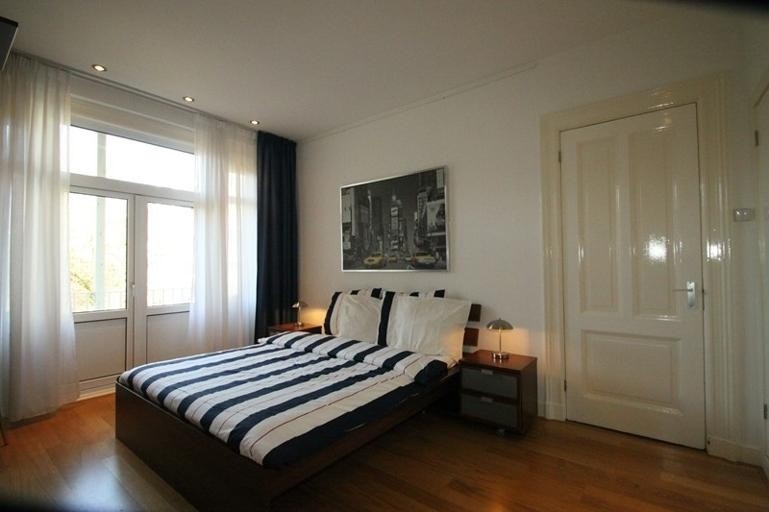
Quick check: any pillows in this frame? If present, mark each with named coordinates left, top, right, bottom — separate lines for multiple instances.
left=324, top=287, right=385, bottom=335
left=383, top=291, right=445, bottom=346
left=329, top=294, right=382, bottom=345
left=385, top=295, right=472, bottom=360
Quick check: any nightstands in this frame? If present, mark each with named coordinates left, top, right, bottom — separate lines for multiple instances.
left=266, top=322, right=322, bottom=336
left=460, top=349, right=536, bottom=436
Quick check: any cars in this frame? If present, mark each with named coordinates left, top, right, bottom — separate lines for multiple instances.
left=411, top=251, right=436, bottom=268
left=388, top=254, right=412, bottom=263
left=363, top=252, right=388, bottom=268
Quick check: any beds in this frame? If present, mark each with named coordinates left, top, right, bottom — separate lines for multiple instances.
left=116, top=304, right=482, bottom=508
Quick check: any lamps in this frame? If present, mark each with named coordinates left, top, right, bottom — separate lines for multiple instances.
left=293, top=302, right=308, bottom=326
left=486, top=317, right=514, bottom=360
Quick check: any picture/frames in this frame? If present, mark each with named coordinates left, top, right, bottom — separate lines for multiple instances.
left=339, top=165, right=450, bottom=274
